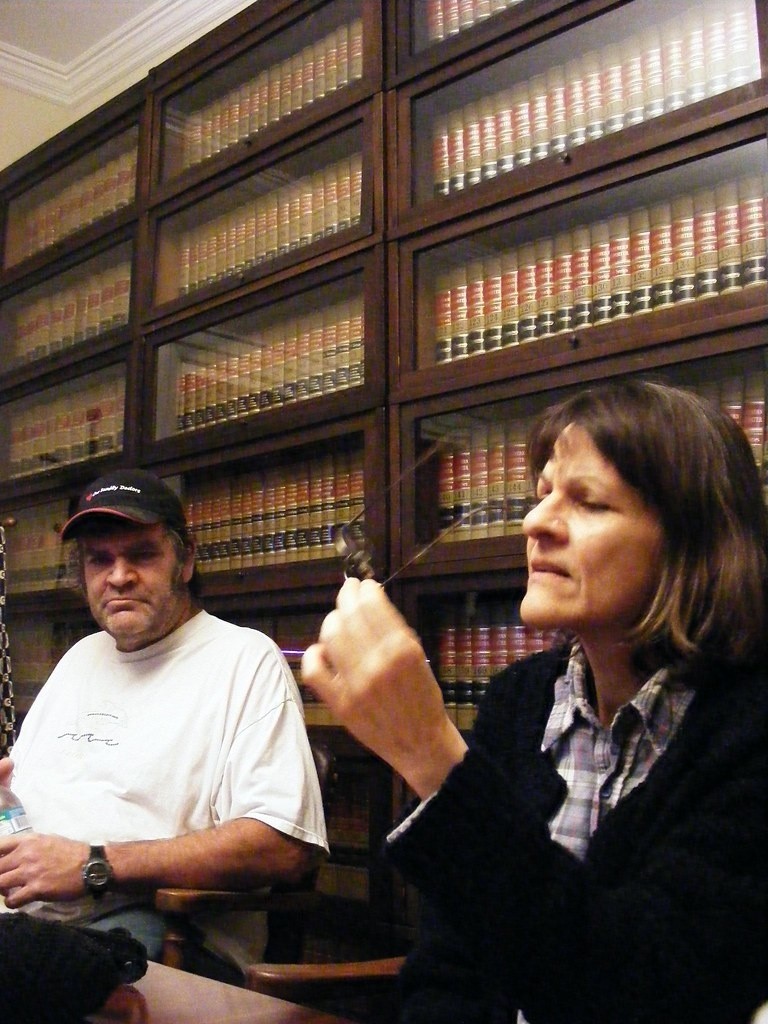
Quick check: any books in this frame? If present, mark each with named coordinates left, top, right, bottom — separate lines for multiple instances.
left=0, top=0, right=768, bottom=968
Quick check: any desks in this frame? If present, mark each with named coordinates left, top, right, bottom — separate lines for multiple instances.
left=125, top=960, right=359, bottom=1023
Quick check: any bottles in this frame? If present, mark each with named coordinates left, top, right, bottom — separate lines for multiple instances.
left=0, top=785, right=48, bottom=914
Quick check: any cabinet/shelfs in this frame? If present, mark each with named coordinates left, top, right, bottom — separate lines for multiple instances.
left=0, top=1, right=767, bottom=1024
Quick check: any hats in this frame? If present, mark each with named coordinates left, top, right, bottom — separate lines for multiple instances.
left=60, top=468, right=186, bottom=540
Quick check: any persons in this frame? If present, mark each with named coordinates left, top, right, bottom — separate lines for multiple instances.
left=300, top=379, right=768, bottom=1024
left=0, top=469, right=330, bottom=989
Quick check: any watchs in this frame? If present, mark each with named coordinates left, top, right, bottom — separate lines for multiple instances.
left=82, top=845, right=113, bottom=900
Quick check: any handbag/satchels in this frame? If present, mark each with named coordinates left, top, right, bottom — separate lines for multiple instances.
left=0, top=911, right=148, bottom=1024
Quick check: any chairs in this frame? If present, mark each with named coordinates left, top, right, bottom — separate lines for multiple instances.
left=156, top=739, right=341, bottom=970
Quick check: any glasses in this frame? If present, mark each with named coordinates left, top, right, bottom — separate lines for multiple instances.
left=331, top=431, right=514, bottom=590
